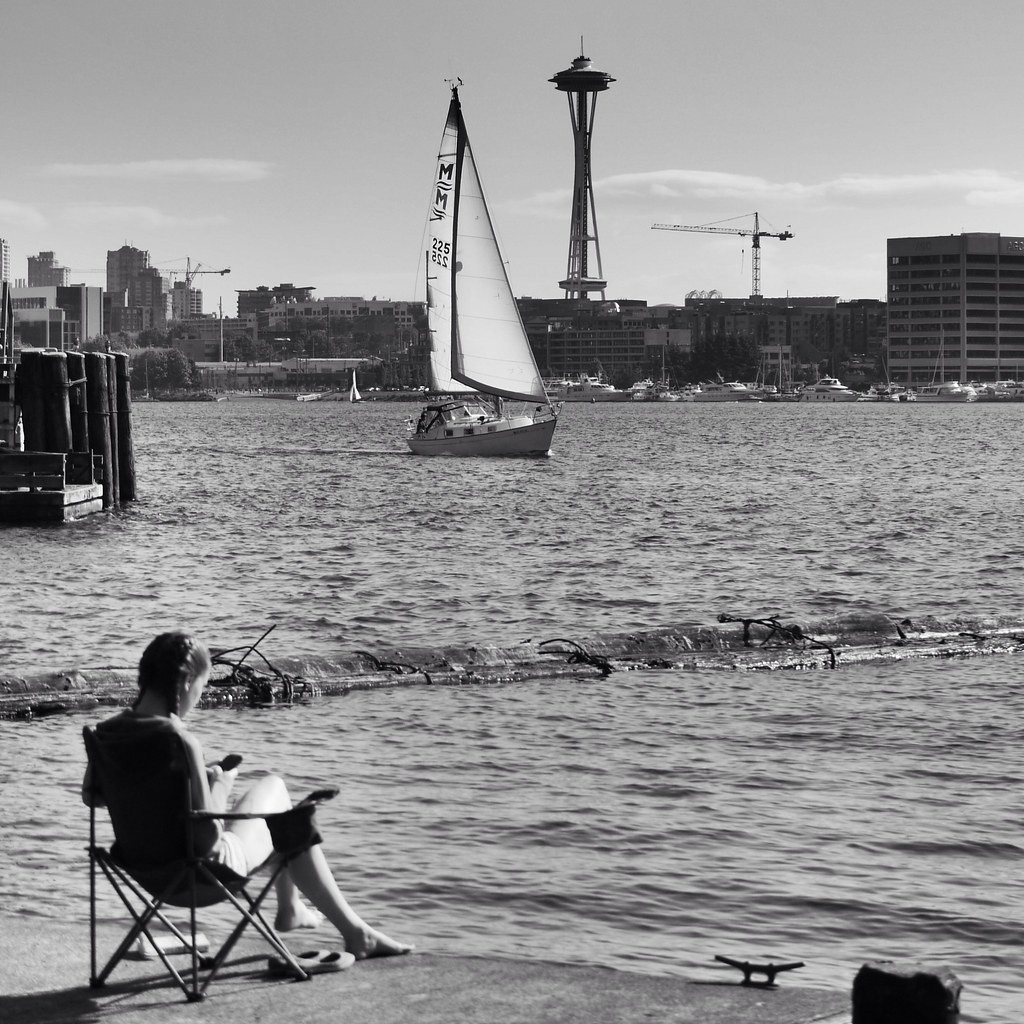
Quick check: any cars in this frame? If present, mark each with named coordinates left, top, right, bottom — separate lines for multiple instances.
left=364, top=385, right=430, bottom=393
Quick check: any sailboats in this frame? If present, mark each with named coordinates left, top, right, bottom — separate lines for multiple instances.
left=350, top=367, right=362, bottom=404
left=405, top=75, right=563, bottom=456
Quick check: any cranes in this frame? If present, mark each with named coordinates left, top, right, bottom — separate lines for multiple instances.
left=160, top=255, right=230, bottom=288
left=651, top=212, right=796, bottom=295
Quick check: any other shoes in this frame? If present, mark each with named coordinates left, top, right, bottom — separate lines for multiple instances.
left=267, top=949, right=331, bottom=977
left=300, top=951, right=355, bottom=973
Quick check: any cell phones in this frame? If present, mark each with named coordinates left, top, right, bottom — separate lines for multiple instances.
left=217, top=754, right=243, bottom=771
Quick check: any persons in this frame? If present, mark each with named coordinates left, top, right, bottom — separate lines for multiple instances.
left=417, top=413, right=428, bottom=433
left=495, top=397, right=504, bottom=417
left=79, top=631, right=415, bottom=958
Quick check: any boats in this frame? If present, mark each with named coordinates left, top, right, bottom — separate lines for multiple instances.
left=542, top=328, right=1024, bottom=403
left=296, top=392, right=321, bottom=402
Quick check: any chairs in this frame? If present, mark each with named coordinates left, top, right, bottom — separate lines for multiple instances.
left=79, top=724, right=341, bottom=1008
